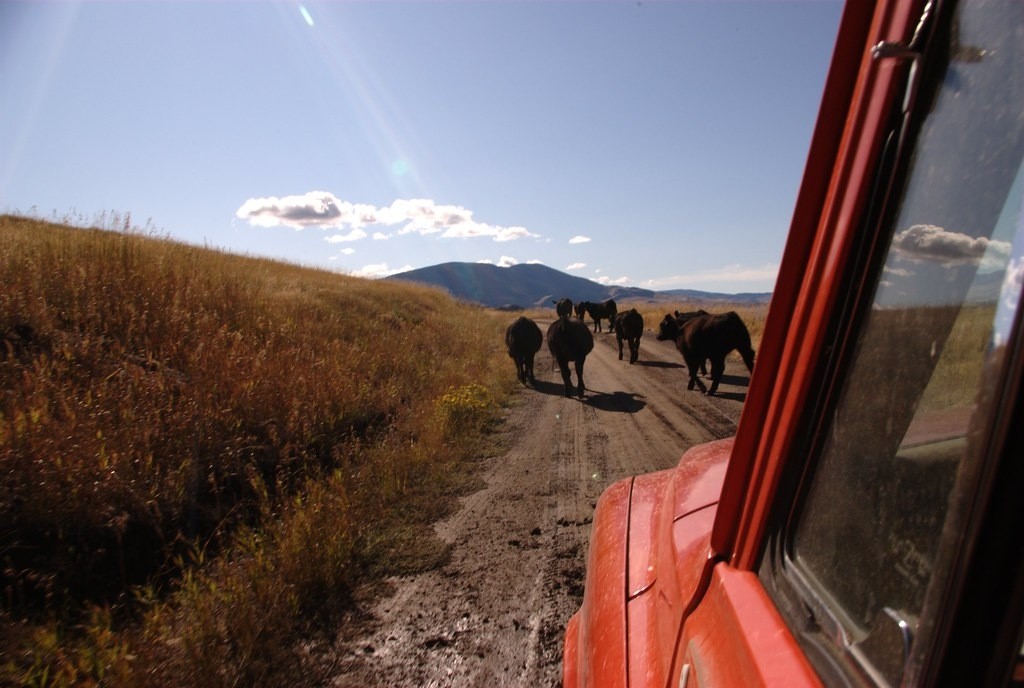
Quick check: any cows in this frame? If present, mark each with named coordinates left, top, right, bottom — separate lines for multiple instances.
left=506, top=299, right=756, bottom=397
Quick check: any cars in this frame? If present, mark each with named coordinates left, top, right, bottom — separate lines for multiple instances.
left=557, top=0, right=1023, bottom=688
